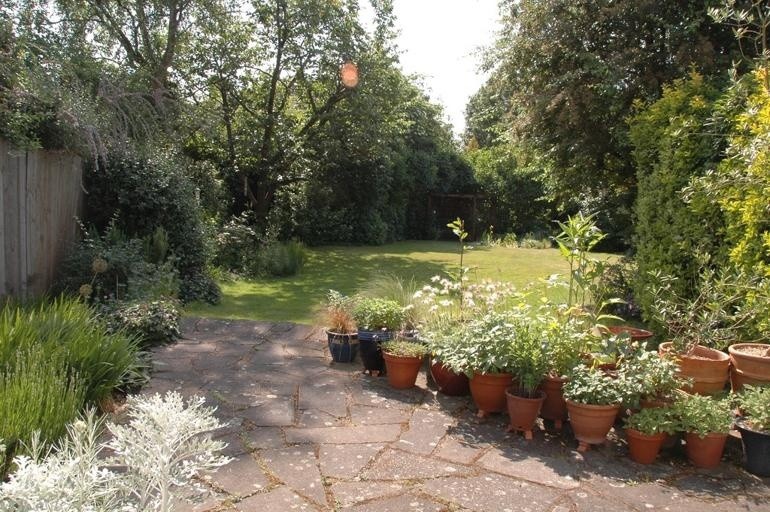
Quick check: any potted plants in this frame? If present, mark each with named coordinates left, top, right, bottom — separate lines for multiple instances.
left=539, top=213, right=769, bottom=452
left=323, top=290, right=361, bottom=362
left=351, top=294, right=406, bottom=376
left=406, top=217, right=546, bottom=441
left=622, top=400, right=677, bottom=465
left=728, top=381, right=769, bottom=477
left=677, top=391, right=732, bottom=471
left=378, top=327, right=428, bottom=389
